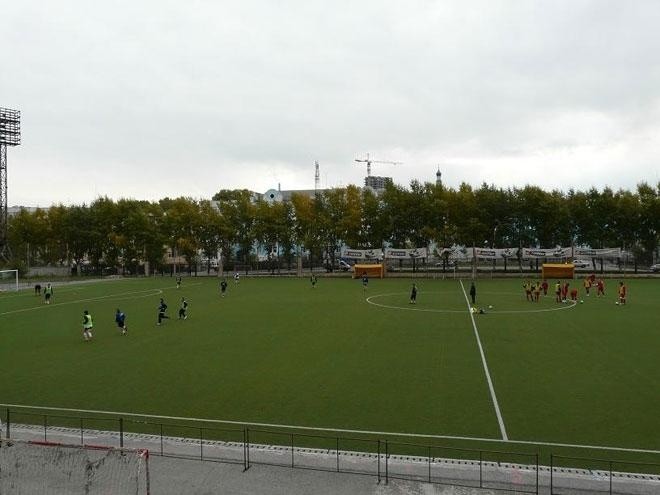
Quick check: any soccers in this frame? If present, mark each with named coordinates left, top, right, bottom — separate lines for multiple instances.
left=489, top=304, right=493, bottom=309
left=615, top=301, right=619, bottom=305
left=580, top=300, right=583, bottom=304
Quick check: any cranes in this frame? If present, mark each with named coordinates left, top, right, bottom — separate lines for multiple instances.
left=353, top=150, right=400, bottom=191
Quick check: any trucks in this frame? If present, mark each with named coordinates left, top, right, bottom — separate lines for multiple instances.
left=322, top=259, right=351, bottom=271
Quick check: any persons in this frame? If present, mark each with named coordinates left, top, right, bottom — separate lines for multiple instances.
left=83, top=311, right=94, bottom=342
left=35, top=284, right=41, bottom=296
left=177, top=297, right=187, bottom=320
left=410, top=284, right=418, bottom=304
left=469, top=281, right=476, bottom=304
left=44, top=283, right=53, bottom=305
left=233, top=272, right=240, bottom=287
left=523, top=272, right=626, bottom=305
left=311, top=274, right=315, bottom=287
left=220, top=278, right=227, bottom=298
left=115, top=307, right=127, bottom=336
left=175, top=272, right=181, bottom=288
left=157, top=299, right=170, bottom=326
left=362, top=271, right=368, bottom=286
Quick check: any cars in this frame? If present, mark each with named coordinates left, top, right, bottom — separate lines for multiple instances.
left=435, top=260, right=455, bottom=268
left=571, top=260, right=590, bottom=268
